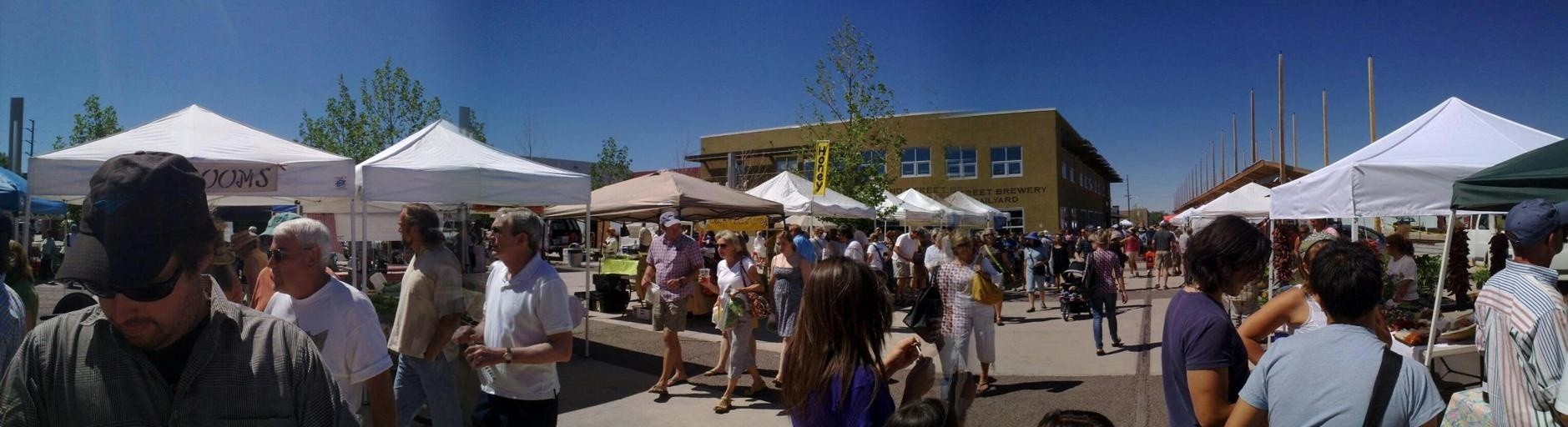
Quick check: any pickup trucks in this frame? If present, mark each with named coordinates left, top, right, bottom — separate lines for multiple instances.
left=1463, top=214, right=1513, bottom=264
left=1393, top=217, right=1424, bottom=231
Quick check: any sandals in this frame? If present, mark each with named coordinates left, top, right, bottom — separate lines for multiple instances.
left=744, top=382, right=768, bottom=394
left=714, top=396, right=733, bottom=412
left=997, top=320, right=1004, bottom=326
left=1026, top=307, right=1035, bottom=312
left=1040, top=303, right=1047, bottom=308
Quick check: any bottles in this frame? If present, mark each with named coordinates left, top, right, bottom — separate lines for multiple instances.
left=466, top=340, right=500, bottom=387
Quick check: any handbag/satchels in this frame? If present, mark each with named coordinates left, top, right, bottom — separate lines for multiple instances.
left=903, top=265, right=944, bottom=344
left=1003, top=269, right=1012, bottom=278
left=748, top=292, right=770, bottom=319
left=1033, top=265, right=1046, bottom=276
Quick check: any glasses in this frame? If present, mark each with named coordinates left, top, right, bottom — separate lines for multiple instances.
left=267, top=248, right=310, bottom=260
left=715, top=243, right=734, bottom=249
left=80, top=259, right=188, bottom=302
left=985, top=233, right=993, bottom=236
left=1385, top=243, right=1390, bottom=247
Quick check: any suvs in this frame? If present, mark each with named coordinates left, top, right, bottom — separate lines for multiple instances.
left=545, top=217, right=582, bottom=256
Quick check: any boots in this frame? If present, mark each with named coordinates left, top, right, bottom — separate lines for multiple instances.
left=704, top=370, right=728, bottom=375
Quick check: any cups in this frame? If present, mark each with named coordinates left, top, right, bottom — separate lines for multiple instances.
left=700, top=269, right=710, bottom=285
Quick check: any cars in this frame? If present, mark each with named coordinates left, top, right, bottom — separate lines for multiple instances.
left=342, top=241, right=388, bottom=263
left=1336, top=224, right=1387, bottom=247
left=19, top=221, right=34, bottom=241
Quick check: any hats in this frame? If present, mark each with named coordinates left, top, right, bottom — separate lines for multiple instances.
left=211, top=244, right=236, bottom=265
left=659, top=211, right=682, bottom=228
left=228, top=231, right=258, bottom=250
left=257, top=211, right=303, bottom=236
left=54, top=150, right=206, bottom=283
left=915, top=227, right=925, bottom=235
left=1085, top=224, right=1098, bottom=231
left=607, top=227, right=616, bottom=235
left=1024, top=231, right=1041, bottom=241
left=1504, top=198, right=1568, bottom=245
left=1044, top=230, right=1049, bottom=237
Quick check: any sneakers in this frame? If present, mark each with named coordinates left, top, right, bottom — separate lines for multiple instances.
left=1172, top=271, right=1178, bottom=276
left=1154, top=284, right=1160, bottom=289
left=1163, top=285, right=1169, bottom=289
left=1129, top=275, right=1135, bottom=278
left=1135, top=272, right=1139, bottom=277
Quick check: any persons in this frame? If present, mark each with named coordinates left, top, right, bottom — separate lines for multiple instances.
left=0, top=148, right=571, bottom=427
left=642, top=212, right=704, bottom=395
left=1161, top=199, right=1568, bottom=425
left=602, top=222, right=1157, bottom=427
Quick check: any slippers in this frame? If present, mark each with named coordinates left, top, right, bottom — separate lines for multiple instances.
left=977, top=382, right=990, bottom=393
left=1111, top=338, right=1121, bottom=345
left=649, top=387, right=669, bottom=396
left=1097, top=350, right=1105, bottom=356
left=668, top=375, right=686, bottom=386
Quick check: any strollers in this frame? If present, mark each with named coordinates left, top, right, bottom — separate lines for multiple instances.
left=1059, top=257, right=1095, bottom=321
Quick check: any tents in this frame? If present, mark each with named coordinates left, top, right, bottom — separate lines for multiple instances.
left=542, top=169, right=787, bottom=259
left=0, top=103, right=356, bottom=288
left=296, top=118, right=590, bottom=358
left=876, top=187, right=1007, bottom=227
left=1159, top=182, right=1270, bottom=235
left=1268, top=96, right=1568, bottom=366
left=692, top=170, right=876, bottom=240
